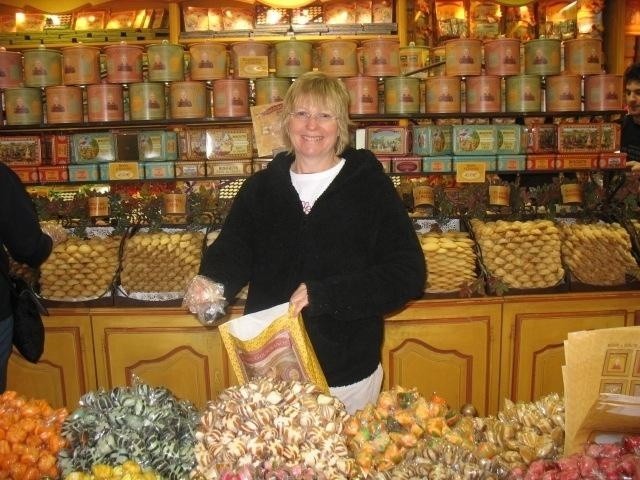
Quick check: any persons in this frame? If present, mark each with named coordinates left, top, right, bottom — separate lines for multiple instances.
left=619, top=60, right=640, bottom=179
left=1, top=159, right=70, bottom=392
left=182, top=72, right=429, bottom=418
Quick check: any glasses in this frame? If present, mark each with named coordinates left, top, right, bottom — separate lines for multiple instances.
left=290, top=111, right=336, bottom=121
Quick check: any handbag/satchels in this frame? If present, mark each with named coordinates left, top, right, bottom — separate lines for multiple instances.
left=9, top=275, right=48, bottom=363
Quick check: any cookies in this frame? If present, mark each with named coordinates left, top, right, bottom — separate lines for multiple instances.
left=0, top=217, right=640, bottom=299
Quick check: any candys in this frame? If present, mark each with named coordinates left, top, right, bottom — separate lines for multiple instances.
left=0, top=379, right=639, bottom=480
